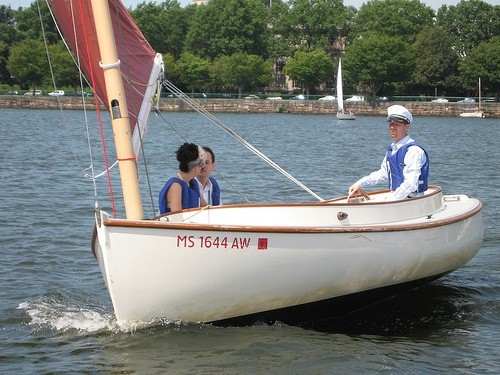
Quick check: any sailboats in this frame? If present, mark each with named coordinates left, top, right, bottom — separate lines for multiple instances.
left=33, top=0, right=485, bottom=335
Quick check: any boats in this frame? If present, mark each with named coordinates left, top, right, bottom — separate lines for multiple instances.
left=336, top=112, right=356, bottom=120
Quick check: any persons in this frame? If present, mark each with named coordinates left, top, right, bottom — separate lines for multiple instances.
left=187, top=147, right=222, bottom=207
left=348, top=105, right=429, bottom=202
left=159, top=143, right=208, bottom=214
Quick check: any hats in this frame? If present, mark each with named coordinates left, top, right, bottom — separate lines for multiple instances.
left=386, top=105, right=413, bottom=124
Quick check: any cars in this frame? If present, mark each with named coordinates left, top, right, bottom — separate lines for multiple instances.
left=4, top=89, right=475, bottom=105
